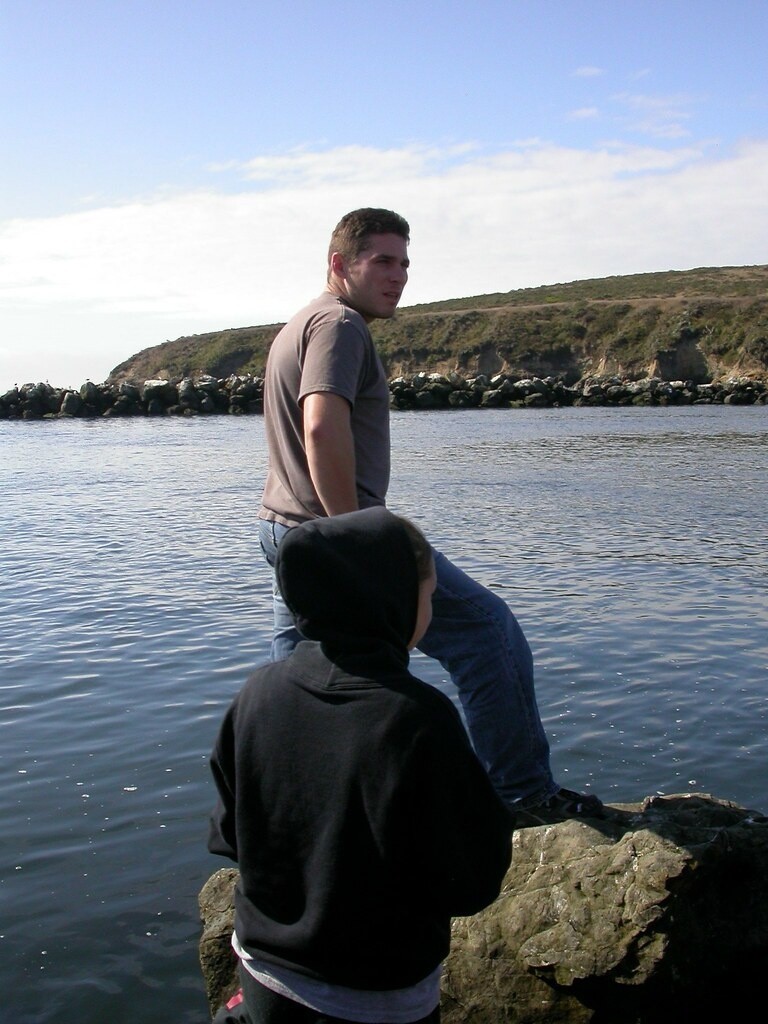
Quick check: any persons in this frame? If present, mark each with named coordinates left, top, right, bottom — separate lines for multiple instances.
left=206, top=506, right=523, bottom=1023
left=255, top=205, right=605, bottom=829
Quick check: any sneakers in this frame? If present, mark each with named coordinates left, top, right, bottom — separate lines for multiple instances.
left=511, top=788, right=603, bottom=829
